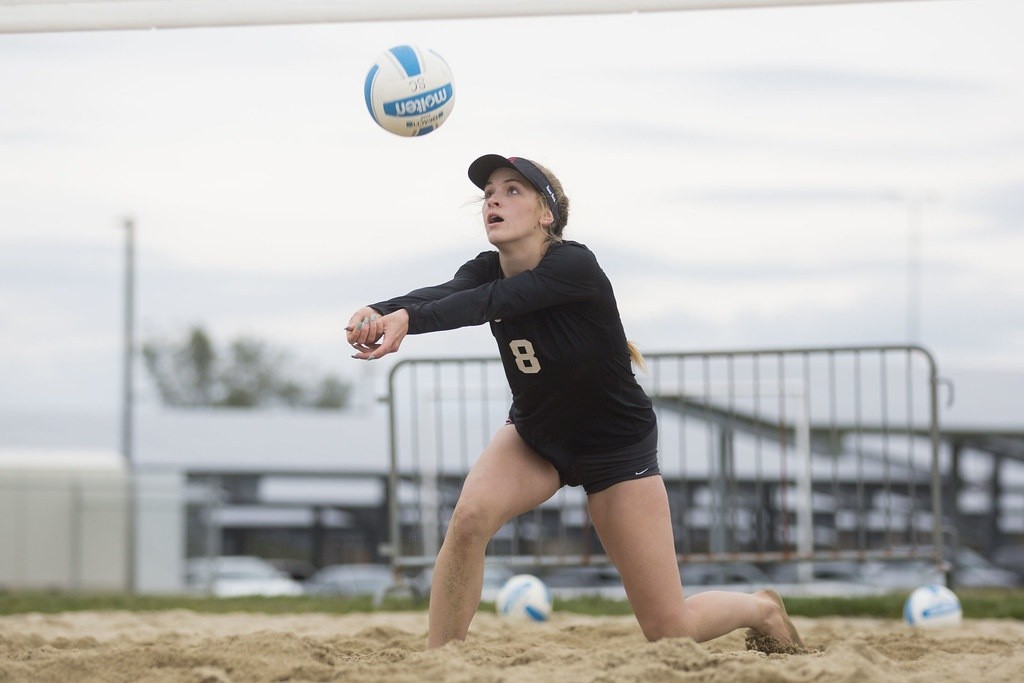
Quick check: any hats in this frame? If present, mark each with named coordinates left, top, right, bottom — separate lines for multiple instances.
left=466, top=153, right=563, bottom=229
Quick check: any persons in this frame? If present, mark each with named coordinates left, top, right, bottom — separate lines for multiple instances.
left=343, top=153, right=806, bottom=659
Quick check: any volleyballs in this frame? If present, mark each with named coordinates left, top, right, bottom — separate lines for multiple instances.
left=364, top=45, right=455, bottom=136
left=496, top=574, right=553, bottom=623
left=900, top=584, right=962, bottom=628
